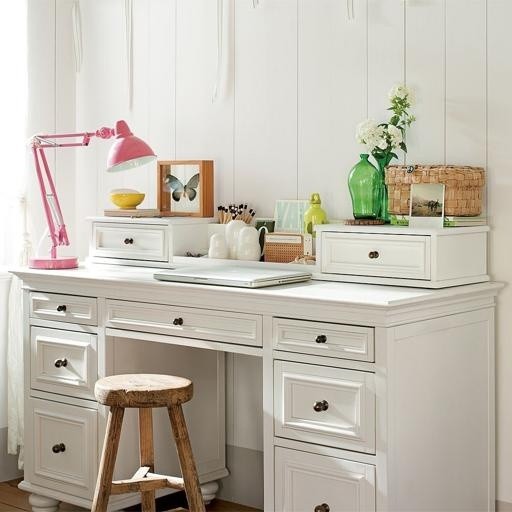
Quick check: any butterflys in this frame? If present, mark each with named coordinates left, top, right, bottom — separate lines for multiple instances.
left=163, top=172, right=200, bottom=203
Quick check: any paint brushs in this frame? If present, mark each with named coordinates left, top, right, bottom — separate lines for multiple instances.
left=217, top=202, right=256, bottom=224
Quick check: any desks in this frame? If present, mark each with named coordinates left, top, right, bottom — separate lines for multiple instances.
left=8, top=217, right=506, bottom=512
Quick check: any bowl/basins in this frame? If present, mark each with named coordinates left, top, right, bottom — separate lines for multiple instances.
left=111, top=192, right=145, bottom=210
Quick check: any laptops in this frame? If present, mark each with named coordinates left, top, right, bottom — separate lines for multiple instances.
left=154, top=270, right=313, bottom=288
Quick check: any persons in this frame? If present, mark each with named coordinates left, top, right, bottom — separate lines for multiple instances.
left=428, top=199, right=440, bottom=214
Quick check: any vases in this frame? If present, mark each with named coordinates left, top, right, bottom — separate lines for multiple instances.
left=370, top=153, right=395, bottom=219
left=347, top=153, right=382, bottom=219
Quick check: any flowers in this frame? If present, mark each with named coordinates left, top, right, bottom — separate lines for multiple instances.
left=353, top=82, right=417, bottom=159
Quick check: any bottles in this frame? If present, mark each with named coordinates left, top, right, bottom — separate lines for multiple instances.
left=304, top=193, right=328, bottom=261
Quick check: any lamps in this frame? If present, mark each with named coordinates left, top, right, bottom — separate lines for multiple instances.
left=26, top=119, right=158, bottom=270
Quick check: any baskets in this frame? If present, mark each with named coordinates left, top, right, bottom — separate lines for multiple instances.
left=263, top=232, right=304, bottom=263
left=384, top=163, right=486, bottom=216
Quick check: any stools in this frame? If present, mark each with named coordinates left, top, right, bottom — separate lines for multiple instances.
left=89, top=371, right=207, bottom=512
